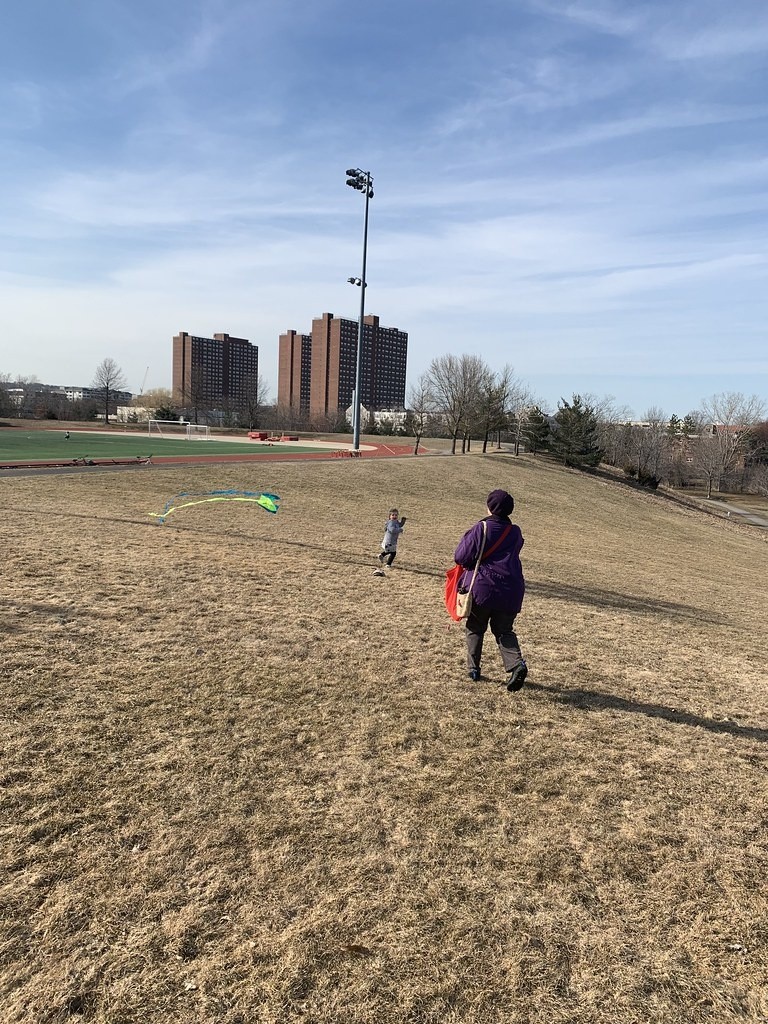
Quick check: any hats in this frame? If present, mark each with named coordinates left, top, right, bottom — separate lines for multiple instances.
left=487, top=489, right=514, bottom=515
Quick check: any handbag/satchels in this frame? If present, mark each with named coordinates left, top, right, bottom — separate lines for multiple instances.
left=446, top=564, right=463, bottom=622
left=456, top=588, right=472, bottom=617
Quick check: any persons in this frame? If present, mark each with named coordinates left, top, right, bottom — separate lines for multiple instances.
left=64, top=431, right=70, bottom=439
left=379, top=509, right=406, bottom=567
left=454, top=489, right=528, bottom=691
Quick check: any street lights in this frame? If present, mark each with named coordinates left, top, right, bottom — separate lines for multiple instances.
left=345, top=167, right=375, bottom=448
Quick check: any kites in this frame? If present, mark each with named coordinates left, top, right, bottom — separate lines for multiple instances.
left=148, top=490, right=281, bottom=522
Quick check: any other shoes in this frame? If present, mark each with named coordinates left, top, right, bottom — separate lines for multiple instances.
left=384, top=564, right=392, bottom=571
left=507, top=664, right=527, bottom=693
left=468, top=668, right=480, bottom=680
left=378, top=554, right=383, bottom=563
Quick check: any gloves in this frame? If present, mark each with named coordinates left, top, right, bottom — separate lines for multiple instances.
left=401, top=517, right=406, bottom=523
left=399, top=529, right=403, bottom=533
